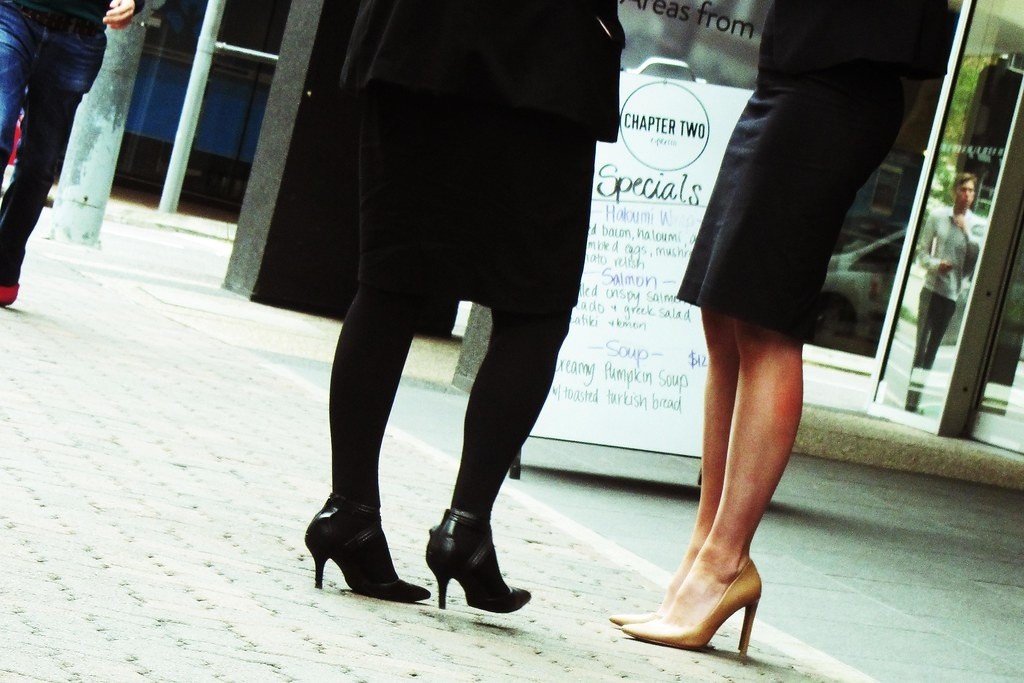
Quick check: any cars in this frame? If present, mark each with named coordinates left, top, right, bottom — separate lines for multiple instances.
left=815, top=214, right=1024, bottom=384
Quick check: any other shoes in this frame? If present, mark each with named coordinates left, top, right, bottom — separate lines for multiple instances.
left=0, top=282, right=20, bottom=305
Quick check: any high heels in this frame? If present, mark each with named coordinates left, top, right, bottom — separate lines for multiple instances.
left=620, top=557, right=762, bottom=657
left=609, top=610, right=668, bottom=627
left=304, top=496, right=431, bottom=601
left=424, top=508, right=531, bottom=613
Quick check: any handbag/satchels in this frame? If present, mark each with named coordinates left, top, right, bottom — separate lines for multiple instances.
left=338, top=0, right=630, bottom=143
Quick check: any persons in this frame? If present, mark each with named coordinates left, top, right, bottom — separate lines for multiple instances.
left=0, top=0, right=145, bottom=305
left=905, top=172, right=978, bottom=412
left=305, top=0, right=622, bottom=613
left=609, top=0, right=942, bottom=654
